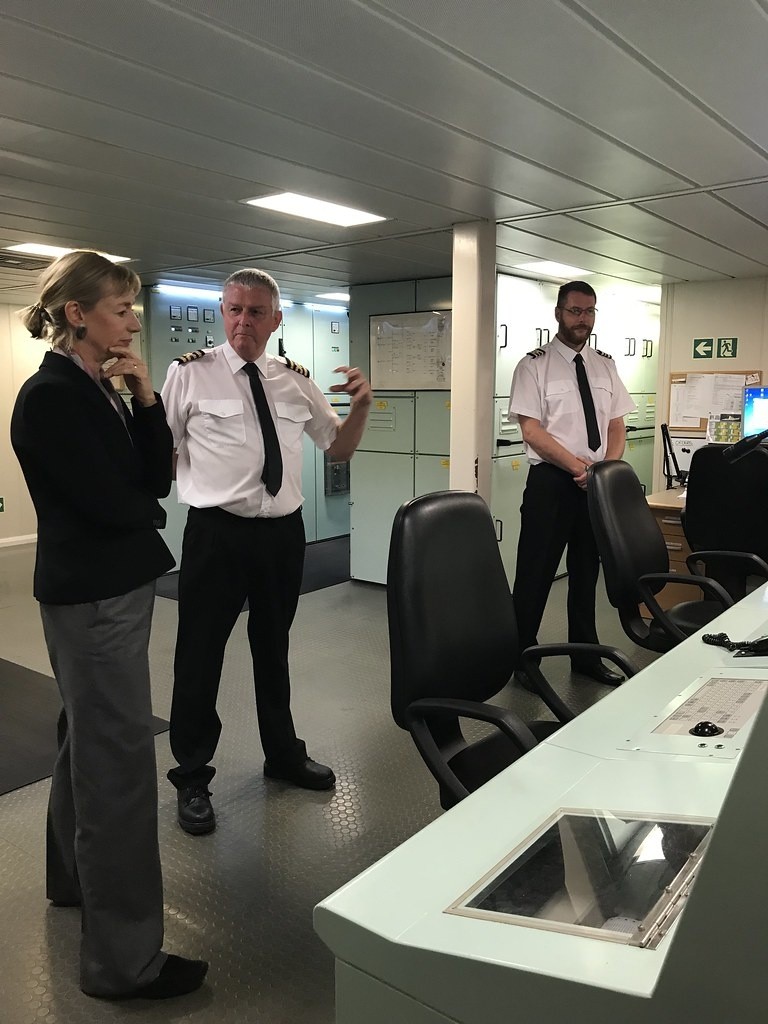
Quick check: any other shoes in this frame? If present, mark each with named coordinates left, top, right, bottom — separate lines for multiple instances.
left=52, top=898, right=82, bottom=907
left=82, top=954, right=209, bottom=1000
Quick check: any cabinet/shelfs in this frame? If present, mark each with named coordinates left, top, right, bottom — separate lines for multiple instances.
left=121, top=279, right=451, bottom=589
left=637, top=485, right=707, bottom=620
left=497, top=274, right=661, bottom=595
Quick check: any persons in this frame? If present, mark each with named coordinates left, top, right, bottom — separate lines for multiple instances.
left=11, top=251, right=210, bottom=1003
left=161, top=268, right=373, bottom=837
left=507, top=280, right=638, bottom=695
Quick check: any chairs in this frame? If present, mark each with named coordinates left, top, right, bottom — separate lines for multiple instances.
left=585, top=458, right=768, bottom=657
left=381, top=487, right=642, bottom=819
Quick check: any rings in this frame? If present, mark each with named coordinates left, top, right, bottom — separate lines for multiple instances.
left=133, top=363, right=137, bottom=368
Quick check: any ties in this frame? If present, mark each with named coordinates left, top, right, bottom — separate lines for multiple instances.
left=573, top=354, right=601, bottom=452
left=242, top=363, right=283, bottom=497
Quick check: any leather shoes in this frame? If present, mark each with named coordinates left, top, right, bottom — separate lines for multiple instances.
left=570, top=660, right=625, bottom=687
left=513, top=668, right=541, bottom=697
left=264, top=754, right=337, bottom=789
left=177, top=782, right=216, bottom=835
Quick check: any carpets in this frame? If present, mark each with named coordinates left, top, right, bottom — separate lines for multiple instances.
left=0, top=658, right=170, bottom=797
left=155, top=535, right=354, bottom=614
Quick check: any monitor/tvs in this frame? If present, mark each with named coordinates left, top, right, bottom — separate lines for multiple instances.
left=741, top=385, right=768, bottom=446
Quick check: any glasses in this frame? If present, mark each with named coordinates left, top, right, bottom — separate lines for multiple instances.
left=557, top=305, right=598, bottom=317
